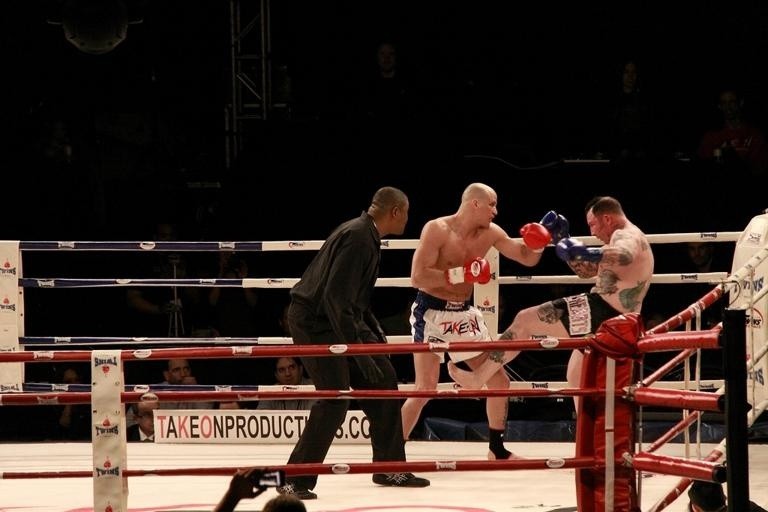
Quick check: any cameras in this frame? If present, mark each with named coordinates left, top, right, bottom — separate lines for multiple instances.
left=255, top=468, right=283, bottom=489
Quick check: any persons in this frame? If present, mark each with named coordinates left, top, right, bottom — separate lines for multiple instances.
left=445, top=195, right=654, bottom=423
left=210, top=467, right=308, bottom=512
left=275, top=184, right=434, bottom=501
left=397, top=181, right=554, bottom=461
left=1, top=3, right=768, bottom=444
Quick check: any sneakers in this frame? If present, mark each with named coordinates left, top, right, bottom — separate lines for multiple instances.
left=372, top=468, right=432, bottom=489
left=275, top=472, right=319, bottom=501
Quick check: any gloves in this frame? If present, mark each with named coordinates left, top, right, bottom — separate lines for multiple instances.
left=374, top=324, right=392, bottom=359
left=445, top=254, right=492, bottom=287
left=537, top=210, right=571, bottom=247
left=517, top=220, right=552, bottom=254
left=555, top=236, right=604, bottom=264
left=352, top=344, right=387, bottom=387
